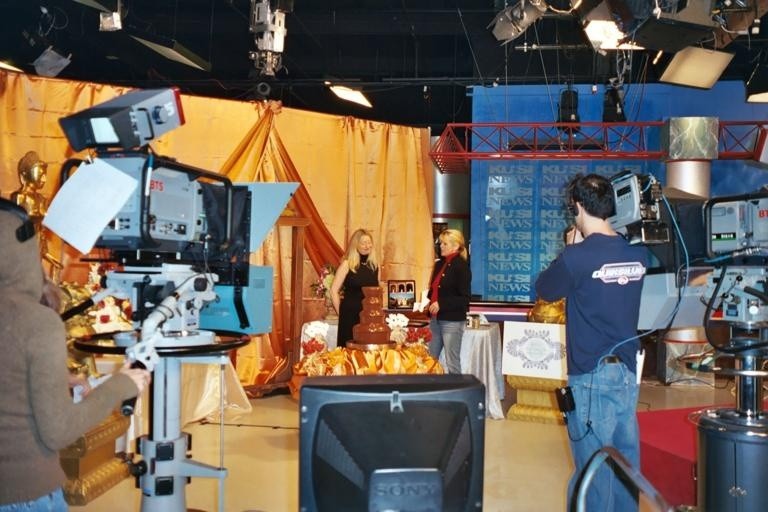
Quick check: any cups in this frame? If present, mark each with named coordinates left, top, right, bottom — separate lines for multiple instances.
left=472, top=319, right=481, bottom=329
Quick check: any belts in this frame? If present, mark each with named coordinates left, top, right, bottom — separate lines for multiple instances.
left=600, top=354, right=623, bottom=365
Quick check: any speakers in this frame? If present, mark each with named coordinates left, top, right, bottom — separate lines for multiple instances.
left=602, top=89, right=627, bottom=123
left=557, top=90, right=581, bottom=136
left=296, top=373, right=486, bottom=512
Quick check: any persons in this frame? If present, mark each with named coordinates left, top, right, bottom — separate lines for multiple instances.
left=329, top=228, right=384, bottom=350
left=533, top=173, right=647, bottom=511
left=423, top=228, right=473, bottom=375
left=1, top=194, right=153, bottom=512
left=10, top=152, right=65, bottom=271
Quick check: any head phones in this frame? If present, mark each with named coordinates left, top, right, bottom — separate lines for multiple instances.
left=571, top=176, right=586, bottom=216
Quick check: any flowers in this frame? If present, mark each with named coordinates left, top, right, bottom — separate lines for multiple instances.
left=313, top=264, right=335, bottom=300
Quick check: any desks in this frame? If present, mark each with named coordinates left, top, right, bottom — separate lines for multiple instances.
left=299, top=319, right=506, bottom=423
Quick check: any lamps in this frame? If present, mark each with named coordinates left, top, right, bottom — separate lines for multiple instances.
left=486, top=2, right=768, bottom=133
left=119, top=0, right=216, bottom=73
